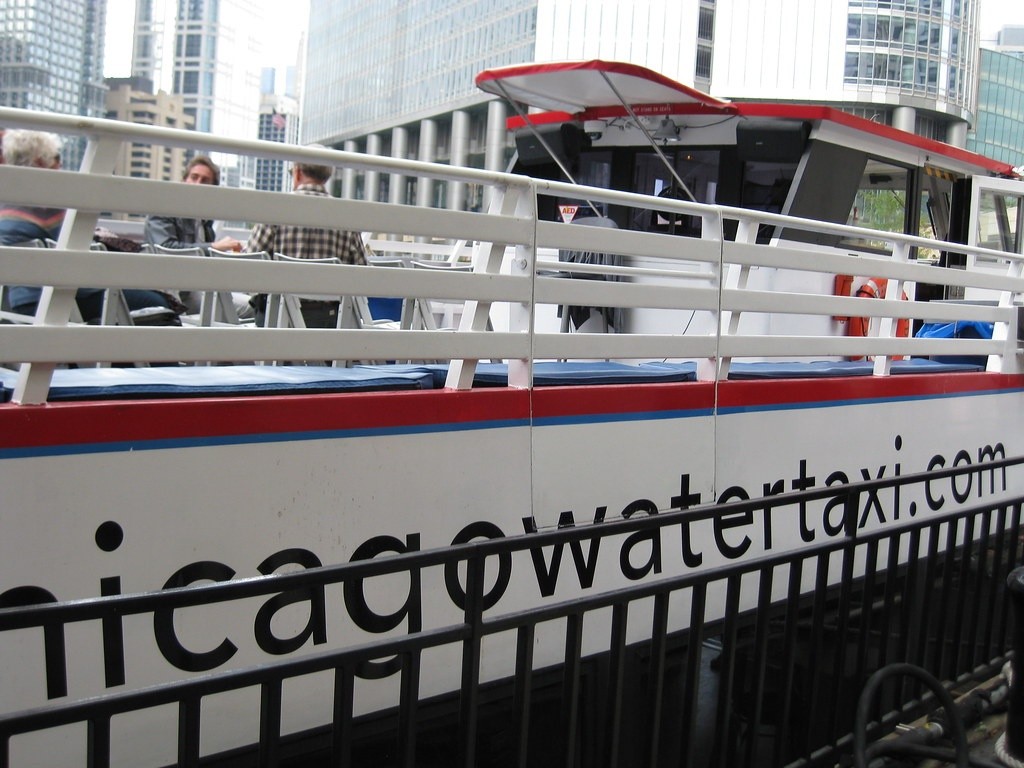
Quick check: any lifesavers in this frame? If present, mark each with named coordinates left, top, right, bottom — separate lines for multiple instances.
left=848, top=276, right=912, bottom=364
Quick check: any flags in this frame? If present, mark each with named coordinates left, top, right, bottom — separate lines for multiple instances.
left=273, top=107, right=287, bottom=130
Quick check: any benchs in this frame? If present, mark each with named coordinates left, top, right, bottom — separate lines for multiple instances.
left=0, top=359, right=985, bottom=403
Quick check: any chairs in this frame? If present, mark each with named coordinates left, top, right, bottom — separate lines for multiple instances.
left=10, top=237, right=503, bottom=368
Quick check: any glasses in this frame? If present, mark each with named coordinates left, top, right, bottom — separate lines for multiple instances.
left=288, top=168, right=296, bottom=176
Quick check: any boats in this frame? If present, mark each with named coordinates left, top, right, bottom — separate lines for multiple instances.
left=0, top=60, right=1023, bottom=767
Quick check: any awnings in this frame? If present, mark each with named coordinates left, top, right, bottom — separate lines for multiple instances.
left=475, top=59, right=736, bottom=217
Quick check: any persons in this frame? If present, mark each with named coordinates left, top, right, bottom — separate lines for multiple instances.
left=241, top=144, right=365, bottom=329
left=144, top=155, right=256, bottom=318
left=0, top=128, right=190, bottom=368
